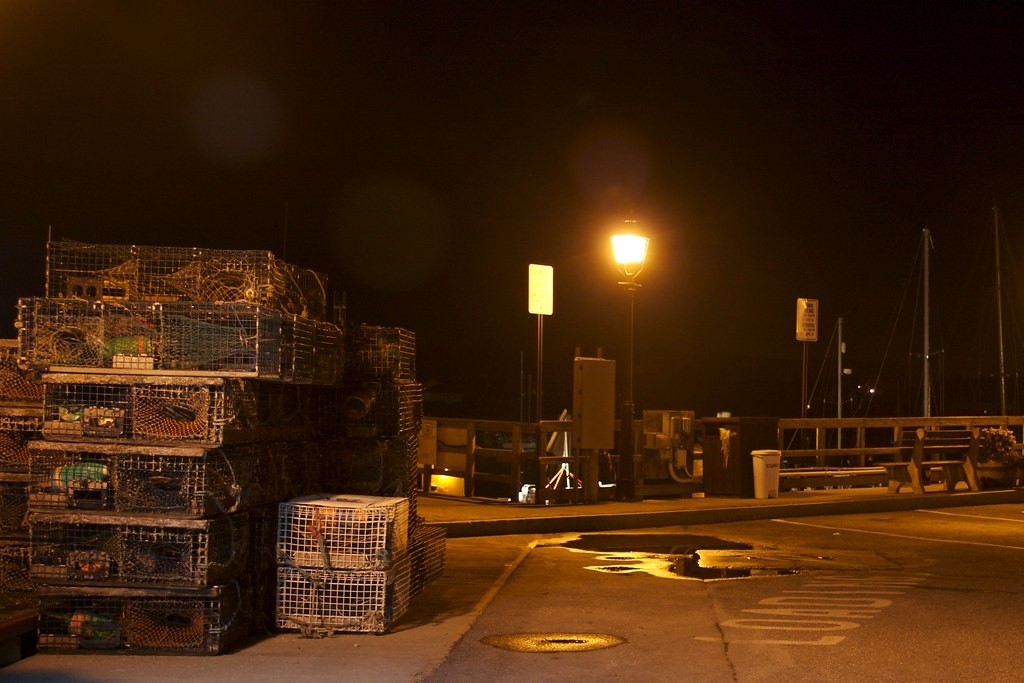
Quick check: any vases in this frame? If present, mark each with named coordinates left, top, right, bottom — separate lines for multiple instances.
left=975, top=462, right=1017, bottom=489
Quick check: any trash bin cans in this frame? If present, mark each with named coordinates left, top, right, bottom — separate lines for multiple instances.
left=750, top=449, right=781, bottom=499
left=700, top=416, right=778, bottom=499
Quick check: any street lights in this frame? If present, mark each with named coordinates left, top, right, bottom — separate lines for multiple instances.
left=609, top=203, right=651, bottom=502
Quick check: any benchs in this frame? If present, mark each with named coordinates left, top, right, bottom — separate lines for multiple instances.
left=879, top=427, right=984, bottom=495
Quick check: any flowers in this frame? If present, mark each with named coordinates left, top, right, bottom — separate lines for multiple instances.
left=978, top=427, right=1024, bottom=462
